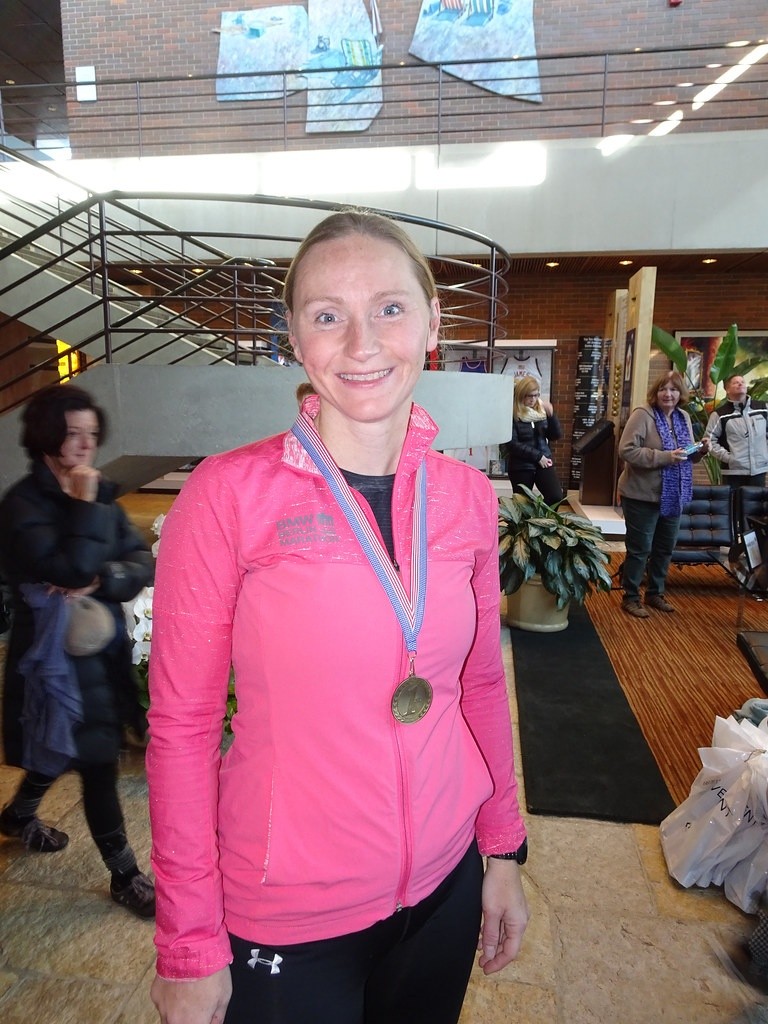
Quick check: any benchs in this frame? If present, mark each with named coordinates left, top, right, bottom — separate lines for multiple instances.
left=670, top=484, right=768, bottom=601
left=736, top=630, right=768, bottom=696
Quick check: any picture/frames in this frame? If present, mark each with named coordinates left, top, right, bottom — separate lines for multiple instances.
left=672, top=330, right=768, bottom=422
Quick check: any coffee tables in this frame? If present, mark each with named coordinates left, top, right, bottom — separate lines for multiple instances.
left=706, top=549, right=768, bottom=601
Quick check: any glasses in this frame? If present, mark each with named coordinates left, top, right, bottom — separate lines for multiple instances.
left=525, top=393, right=541, bottom=399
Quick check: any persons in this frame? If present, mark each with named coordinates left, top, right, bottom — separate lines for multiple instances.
left=497, top=375, right=563, bottom=508
left=0, top=385, right=157, bottom=921
left=702, top=375, right=768, bottom=486
left=616, top=371, right=713, bottom=618
left=146, top=209, right=532, bottom=1024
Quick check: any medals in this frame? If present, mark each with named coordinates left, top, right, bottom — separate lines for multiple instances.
left=391, top=659, right=433, bottom=725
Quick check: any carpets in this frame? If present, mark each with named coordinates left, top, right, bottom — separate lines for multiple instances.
left=0, top=622, right=768, bottom=1022
left=510, top=596, right=677, bottom=822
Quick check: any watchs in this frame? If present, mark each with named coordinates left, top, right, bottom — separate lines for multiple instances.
left=489, top=835, right=530, bottom=865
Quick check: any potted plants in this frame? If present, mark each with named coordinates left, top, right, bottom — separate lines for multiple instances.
left=497, top=483, right=614, bottom=631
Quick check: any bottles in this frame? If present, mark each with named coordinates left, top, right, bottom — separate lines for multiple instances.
left=676, top=442, right=704, bottom=457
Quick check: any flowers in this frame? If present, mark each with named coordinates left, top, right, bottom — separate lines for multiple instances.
left=118, top=514, right=239, bottom=722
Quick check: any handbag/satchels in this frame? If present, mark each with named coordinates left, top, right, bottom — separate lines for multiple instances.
left=499, top=443, right=509, bottom=460
left=61, top=593, right=115, bottom=656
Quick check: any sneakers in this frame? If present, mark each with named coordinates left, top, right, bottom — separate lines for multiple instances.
left=643, top=593, right=675, bottom=611
left=621, top=600, right=649, bottom=618
left=111, top=874, right=157, bottom=917
left=0, top=803, right=69, bottom=852
